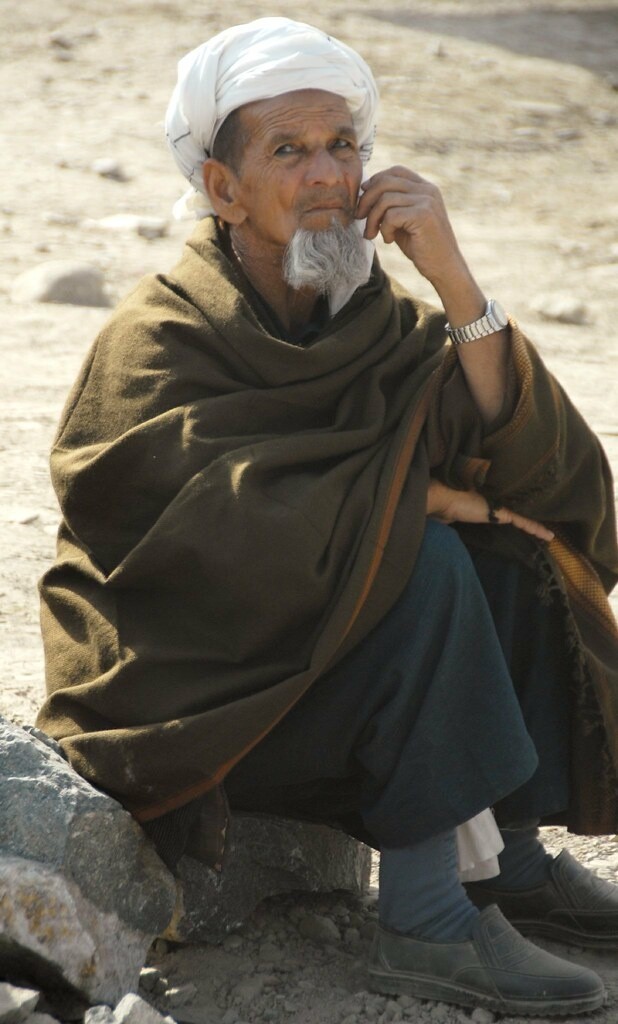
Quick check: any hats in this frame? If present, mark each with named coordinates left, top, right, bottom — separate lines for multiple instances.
left=164, top=16, right=379, bottom=193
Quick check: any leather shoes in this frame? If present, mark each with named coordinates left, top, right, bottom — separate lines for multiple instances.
left=463, top=847, right=618, bottom=946
left=365, top=901, right=607, bottom=1017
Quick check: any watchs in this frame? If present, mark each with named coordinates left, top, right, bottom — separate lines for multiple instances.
left=444, top=298, right=510, bottom=345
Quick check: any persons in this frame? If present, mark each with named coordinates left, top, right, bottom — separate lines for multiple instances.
left=30, top=15, right=618, bottom=1018
